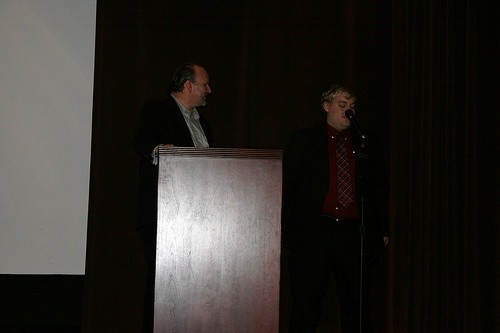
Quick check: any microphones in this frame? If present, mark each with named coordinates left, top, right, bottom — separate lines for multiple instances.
left=345, top=109, right=366, bottom=139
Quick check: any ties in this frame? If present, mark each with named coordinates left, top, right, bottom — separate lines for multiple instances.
left=334, top=134, right=354, bottom=208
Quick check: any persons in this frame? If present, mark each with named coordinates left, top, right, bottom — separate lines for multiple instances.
left=133, top=62, right=218, bottom=333
left=281, top=85, right=390, bottom=332
